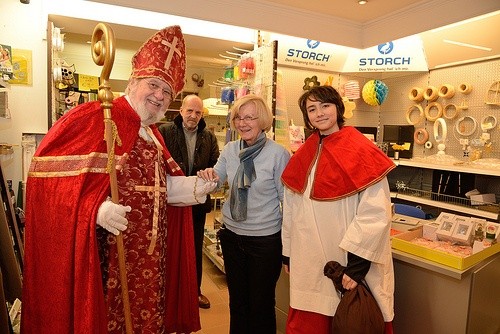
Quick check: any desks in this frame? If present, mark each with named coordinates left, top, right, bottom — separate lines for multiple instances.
left=275, top=214, right=500, bottom=334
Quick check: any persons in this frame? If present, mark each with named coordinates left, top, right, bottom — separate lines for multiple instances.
left=196, top=94, right=292, bottom=334
left=157, top=94, right=221, bottom=310
left=20, top=26, right=218, bottom=334
left=280, top=86, right=396, bottom=334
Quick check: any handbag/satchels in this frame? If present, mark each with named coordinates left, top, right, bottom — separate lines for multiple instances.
left=323, top=259, right=385, bottom=334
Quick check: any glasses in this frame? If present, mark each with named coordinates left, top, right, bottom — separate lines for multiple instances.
left=234, top=115, right=258, bottom=121
left=143, top=79, right=172, bottom=100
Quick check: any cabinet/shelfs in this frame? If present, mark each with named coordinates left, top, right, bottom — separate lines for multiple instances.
left=388, top=157, right=500, bottom=223
left=202, top=107, right=228, bottom=274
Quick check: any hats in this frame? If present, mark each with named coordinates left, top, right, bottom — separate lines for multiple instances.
left=131, top=25, right=187, bottom=100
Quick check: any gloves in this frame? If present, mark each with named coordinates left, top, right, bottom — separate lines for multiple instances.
left=196, top=178, right=218, bottom=198
left=96, top=199, right=132, bottom=234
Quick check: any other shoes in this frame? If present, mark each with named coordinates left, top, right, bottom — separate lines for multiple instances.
left=198, top=295, right=210, bottom=308
